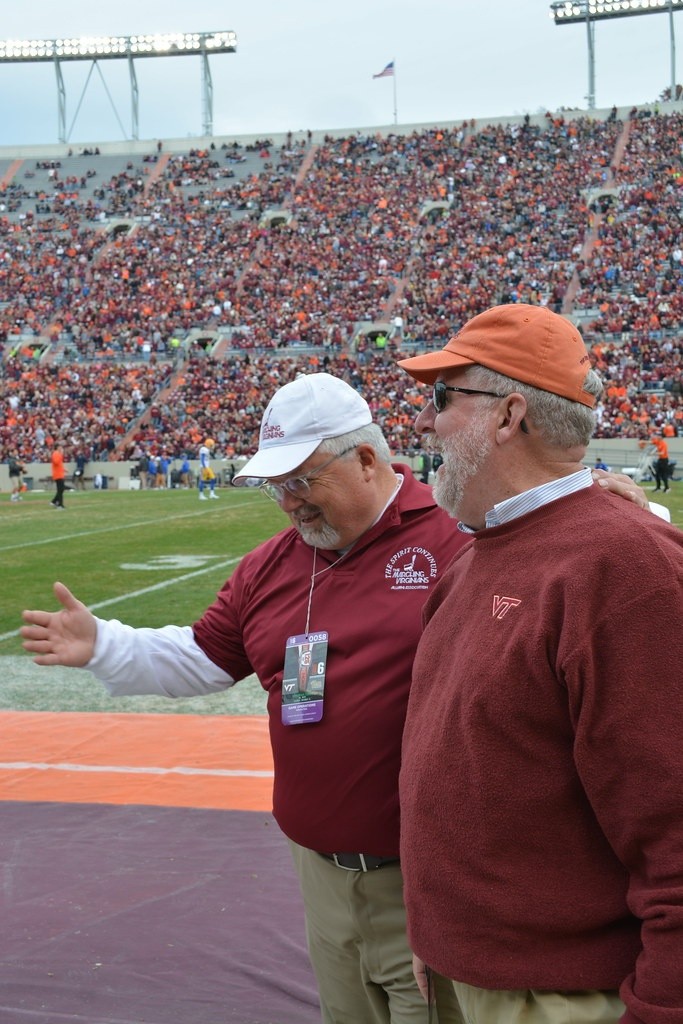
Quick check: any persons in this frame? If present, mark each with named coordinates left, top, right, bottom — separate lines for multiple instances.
left=639, top=435, right=671, bottom=493
left=0, top=105, right=683, bottom=462
left=136, top=451, right=171, bottom=490
left=595, top=458, right=607, bottom=471
left=397, top=303, right=683, bottom=1023
left=21, top=371, right=473, bottom=1022
left=50, top=445, right=65, bottom=509
left=9, top=448, right=27, bottom=501
left=419, top=453, right=443, bottom=483
left=198, top=438, right=220, bottom=500
left=72, top=451, right=89, bottom=490
left=181, top=459, right=192, bottom=488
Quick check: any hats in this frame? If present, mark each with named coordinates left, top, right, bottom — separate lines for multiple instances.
left=232, top=373, right=372, bottom=486
left=396, top=304, right=597, bottom=410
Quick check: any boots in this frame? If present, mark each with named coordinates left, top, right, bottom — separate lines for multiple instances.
left=199, top=492, right=208, bottom=500
left=209, top=490, right=219, bottom=499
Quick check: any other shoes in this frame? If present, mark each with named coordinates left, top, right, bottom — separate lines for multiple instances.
left=11, top=492, right=22, bottom=501
left=57, top=505, right=64, bottom=509
left=50, top=502, right=57, bottom=508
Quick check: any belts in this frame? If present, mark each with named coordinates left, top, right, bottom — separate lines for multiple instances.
left=318, top=852, right=400, bottom=872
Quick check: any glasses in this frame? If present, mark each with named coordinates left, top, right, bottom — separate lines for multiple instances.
left=432, top=382, right=528, bottom=433
left=258, top=445, right=358, bottom=502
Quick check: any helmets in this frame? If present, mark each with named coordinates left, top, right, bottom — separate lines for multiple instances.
left=205, top=439, right=214, bottom=447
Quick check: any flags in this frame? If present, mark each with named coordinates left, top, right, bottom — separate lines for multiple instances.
left=375, top=62, right=395, bottom=78
